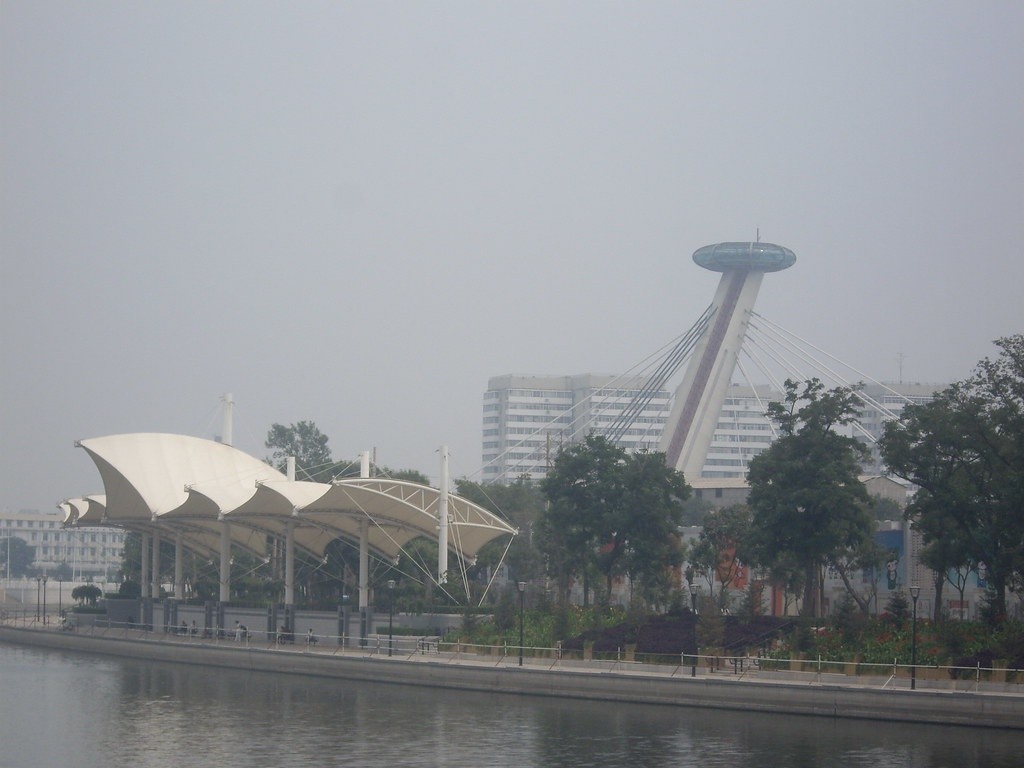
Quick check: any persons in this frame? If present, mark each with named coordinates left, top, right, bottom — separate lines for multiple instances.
left=239, top=625, right=246, bottom=636
left=233, top=621, right=241, bottom=642
left=190, top=621, right=198, bottom=634
left=180, top=621, right=187, bottom=634
left=307, top=629, right=313, bottom=644
left=280, top=626, right=285, bottom=644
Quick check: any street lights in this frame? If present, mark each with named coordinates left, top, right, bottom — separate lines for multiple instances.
left=37, top=575, right=42, bottom=622
left=689, top=583, right=701, bottom=678
left=42, top=575, right=48, bottom=624
left=386, top=579, right=397, bottom=657
left=58, top=574, right=63, bottom=617
left=518, top=581, right=527, bottom=666
left=910, top=585, right=922, bottom=689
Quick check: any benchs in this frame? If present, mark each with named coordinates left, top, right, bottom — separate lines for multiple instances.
left=173, top=626, right=319, bottom=645
